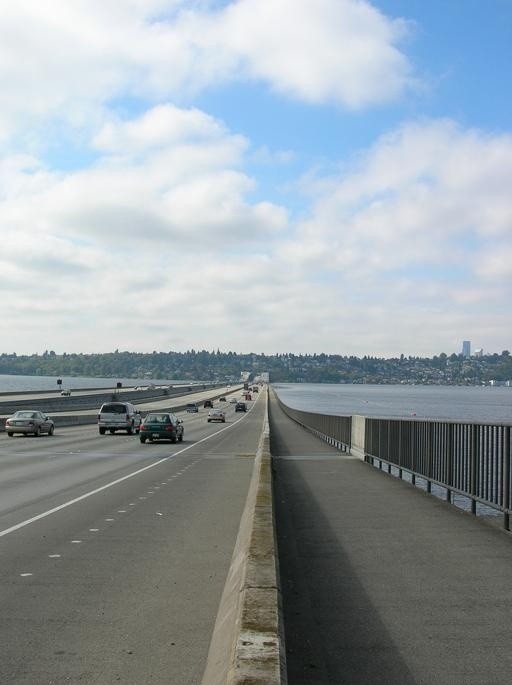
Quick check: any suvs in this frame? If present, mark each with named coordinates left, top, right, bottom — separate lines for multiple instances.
left=98, top=402, right=143, bottom=436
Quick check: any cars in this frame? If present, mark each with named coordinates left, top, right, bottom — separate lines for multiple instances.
left=207, top=409, right=225, bottom=423
left=187, top=401, right=199, bottom=413
left=235, top=403, right=248, bottom=412
left=60, top=388, right=72, bottom=396
left=204, top=399, right=213, bottom=408
left=219, top=396, right=226, bottom=402
left=139, top=413, right=185, bottom=444
left=6, top=410, right=55, bottom=437
left=230, top=383, right=252, bottom=403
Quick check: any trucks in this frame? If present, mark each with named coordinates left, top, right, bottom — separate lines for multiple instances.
left=253, top=386, right=258, bottom=393
